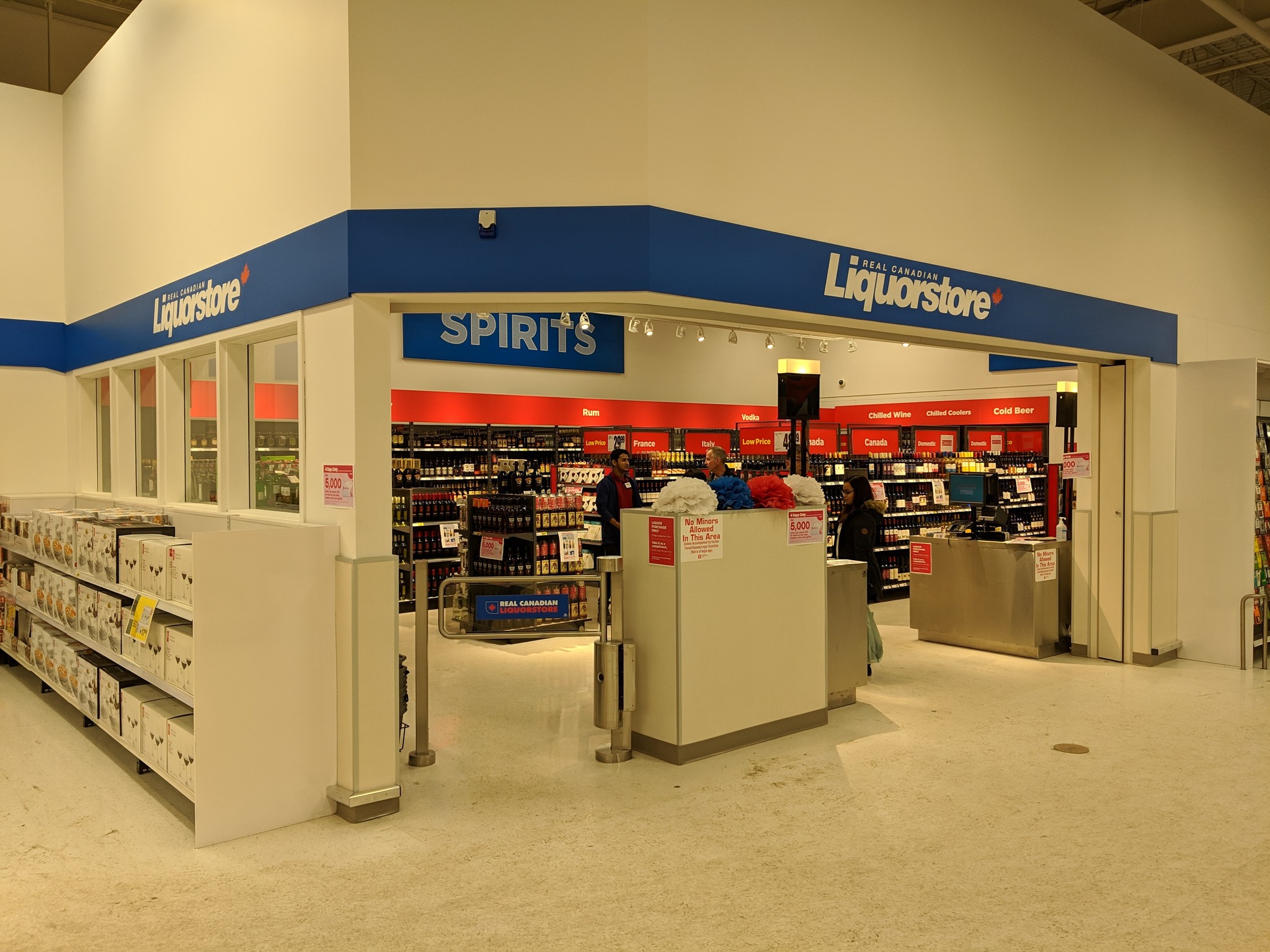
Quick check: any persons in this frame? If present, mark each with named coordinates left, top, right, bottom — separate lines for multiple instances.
left=683, top=469, right=706, bottom=482
left=832, top=474, right=886, bottom=605
left=704, top=446, right=738, bottom=482
left=785, top=445, right=830, bottom=537
left=596, top=449, right=643, bottom=626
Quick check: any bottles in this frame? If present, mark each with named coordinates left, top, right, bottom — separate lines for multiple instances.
left=392, top=424, right=1045, bottom=624
left=190, top=431, right=216, bottom=503
left=255, top=432, right=299, bottom=506
left=141, top=460, right=156, bottom=498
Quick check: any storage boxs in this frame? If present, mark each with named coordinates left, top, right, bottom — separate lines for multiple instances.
left=0, top=509, right=194, bottom=790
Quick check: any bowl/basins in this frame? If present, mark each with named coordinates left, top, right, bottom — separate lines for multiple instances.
left=34, top=519, right=78, bottom=697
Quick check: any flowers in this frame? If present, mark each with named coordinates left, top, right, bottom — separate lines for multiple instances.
left=651, top=474, right=825, bottom=517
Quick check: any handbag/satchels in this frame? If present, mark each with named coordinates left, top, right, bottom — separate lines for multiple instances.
left=867, top=603, right=883, bottom=664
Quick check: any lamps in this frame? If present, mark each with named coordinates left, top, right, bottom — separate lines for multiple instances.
left=777, top=359, right=820, bottom=477
left=558, top=312, right=857, bottom=353
left=1055, top=381, right=1077, bottom=529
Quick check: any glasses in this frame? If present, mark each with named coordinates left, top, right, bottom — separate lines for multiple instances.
left=841, top=489, right=854, bottom=494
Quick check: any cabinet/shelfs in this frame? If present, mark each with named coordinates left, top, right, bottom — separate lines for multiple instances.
left=0, top=491, right=338, bottom=849
left=144, top=418, right=1048, bottom=636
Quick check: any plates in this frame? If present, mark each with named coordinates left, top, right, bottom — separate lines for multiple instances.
left=78, top=553, right=120, bottom=734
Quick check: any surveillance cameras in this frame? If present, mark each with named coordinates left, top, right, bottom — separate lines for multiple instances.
left=839, top=379, right=845, bottom=387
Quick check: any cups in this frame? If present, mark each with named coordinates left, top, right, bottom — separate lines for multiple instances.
left=79, top=530, right=117, bottom=717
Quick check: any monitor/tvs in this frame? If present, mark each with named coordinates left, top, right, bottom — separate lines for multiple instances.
left=949, top=471, right=1000, bottom=537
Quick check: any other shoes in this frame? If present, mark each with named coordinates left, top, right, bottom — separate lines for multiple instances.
left=867, top=664, right=872, bottom=676
